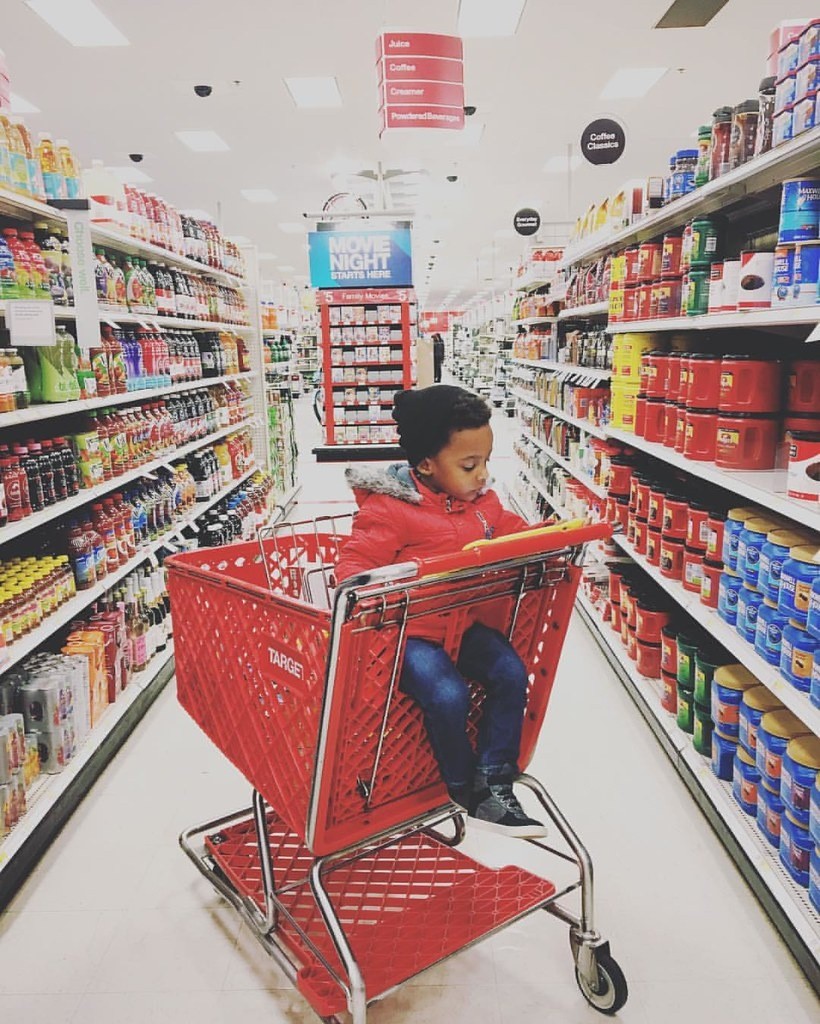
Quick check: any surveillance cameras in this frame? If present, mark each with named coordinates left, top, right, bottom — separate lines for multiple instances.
left=194, top=86, right=212, bottom=97
left=447, top=176, right=458, bottom=182
left=464, top=107, right=476, bottom=116
left=434, top=240, right=439, bottom=243
left=129, top=154, right=143, bottom=162
left=426, top=256, right=435, bottom=284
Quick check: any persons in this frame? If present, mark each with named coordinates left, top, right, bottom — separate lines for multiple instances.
left=431, top=335, right=444, bottom=383
left=435, top=333, right=445, bottom=382
left=334, top=384, right=593, bottom=839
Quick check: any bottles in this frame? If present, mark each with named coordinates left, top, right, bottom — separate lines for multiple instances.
left=1, top=109, right=296, bottom=839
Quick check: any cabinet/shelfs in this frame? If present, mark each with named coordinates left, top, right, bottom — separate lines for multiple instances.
left=0, top=125, right=820, bottom=1002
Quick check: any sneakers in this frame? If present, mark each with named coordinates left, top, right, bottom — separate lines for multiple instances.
left=466, top=773, right=546, bottom=839
left=447, top=785, right=489, bottom=814
